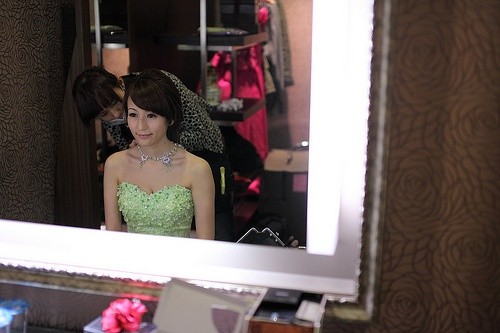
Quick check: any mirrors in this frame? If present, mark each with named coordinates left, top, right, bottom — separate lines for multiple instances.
left=0, top=0, right=400, bottom=325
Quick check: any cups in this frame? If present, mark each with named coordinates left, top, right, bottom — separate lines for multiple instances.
left=0, top=300, right=28, bottom=333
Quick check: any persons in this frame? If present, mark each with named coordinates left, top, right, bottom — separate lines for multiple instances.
left=71, top=66, right=235, bottom=241
left=103, top=68, right=215, bottom=241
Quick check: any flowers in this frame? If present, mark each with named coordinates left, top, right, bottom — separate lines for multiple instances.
left=100, top=296, right=148, bottom=333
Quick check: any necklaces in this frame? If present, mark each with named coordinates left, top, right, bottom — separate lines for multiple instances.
left=137, top=141, right=185, bottom=168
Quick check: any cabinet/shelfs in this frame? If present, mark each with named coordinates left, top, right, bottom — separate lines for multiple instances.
left=178, top=0, right=268, bottom=239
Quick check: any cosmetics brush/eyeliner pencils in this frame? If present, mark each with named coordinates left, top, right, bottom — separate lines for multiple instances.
left=285, top=236, right=299, bottom=248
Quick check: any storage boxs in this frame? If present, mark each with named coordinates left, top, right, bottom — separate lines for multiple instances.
left=259, top=147, right=309, bottom=248
